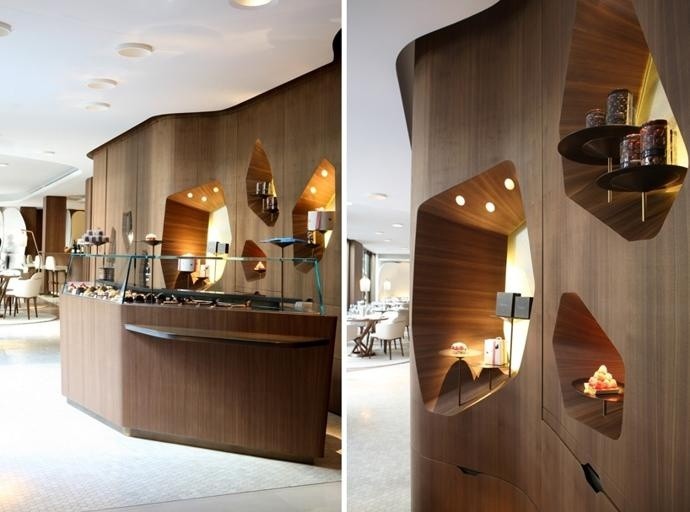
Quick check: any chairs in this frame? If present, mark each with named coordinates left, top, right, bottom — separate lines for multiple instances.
left=3, top=255, right=68, bottom=320
left=347, top=298, right=409, bottom=360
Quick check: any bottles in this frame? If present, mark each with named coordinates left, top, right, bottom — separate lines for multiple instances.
left=71, top=239, right=83, bottom=253
left=640, top=119, right=668, bottom=168
left=583, top=108, right=605, bottom=130
left=603, top=88, right=633, bottom=127
left=619, top=131, right=642, bottom=168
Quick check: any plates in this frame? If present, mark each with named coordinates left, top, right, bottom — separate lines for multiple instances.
left=581, top=383, right=620, bottom=397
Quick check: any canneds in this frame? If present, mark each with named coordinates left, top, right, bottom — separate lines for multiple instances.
left=619, top=134, right=640, bottom=168
left=606, top=89, right=633, bottom=125
left=639, top=119, right=678, bottom=165
left=585, top=109, right=606, bottom=128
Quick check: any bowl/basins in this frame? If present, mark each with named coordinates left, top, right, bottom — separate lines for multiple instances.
left=123, top=299, right=167, bottom=303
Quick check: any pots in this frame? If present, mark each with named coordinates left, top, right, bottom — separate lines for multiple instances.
left=165, top=295, right=252, bottom=309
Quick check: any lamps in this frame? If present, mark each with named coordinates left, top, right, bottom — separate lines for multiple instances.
left=359, top=244, right=370, bottom=291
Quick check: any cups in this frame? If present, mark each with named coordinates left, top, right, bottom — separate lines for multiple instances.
left=82, top=230, right=109, bottom=242
left=255, top=181, right=277, bottom=208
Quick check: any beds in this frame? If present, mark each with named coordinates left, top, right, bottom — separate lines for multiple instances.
left=0, top=274, right=20, bottom=318
